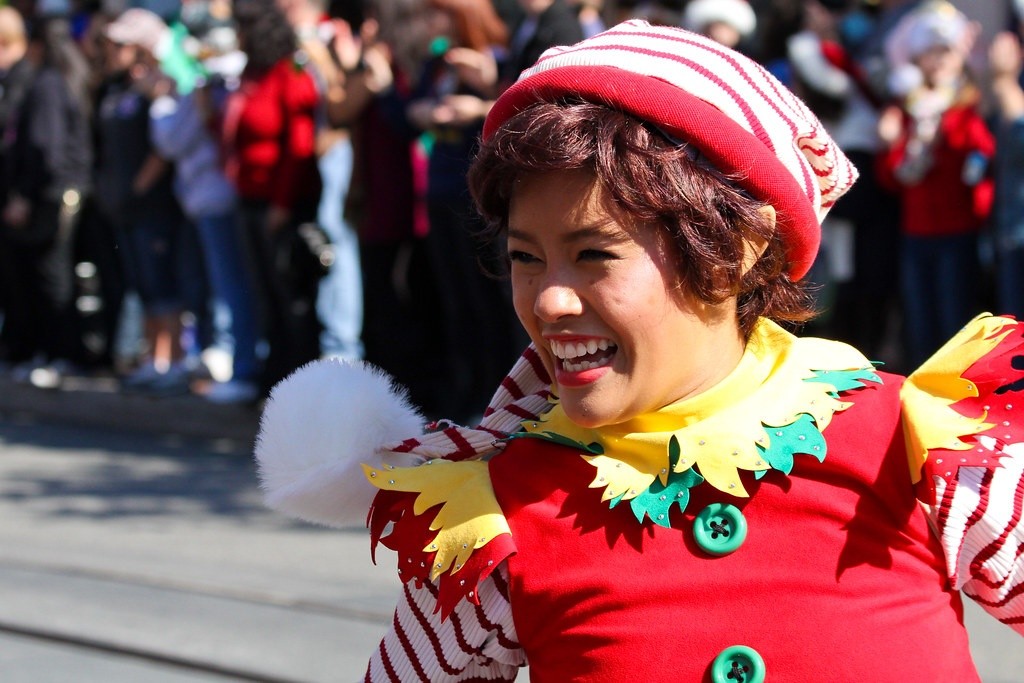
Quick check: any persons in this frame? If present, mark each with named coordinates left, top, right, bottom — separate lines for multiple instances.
left=255, top=18, right=1024, bottom=683
left=1, top=0, right=1024, bottom=423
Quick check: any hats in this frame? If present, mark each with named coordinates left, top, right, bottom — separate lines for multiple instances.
left=101, top=9, right=168, bottom=50
left=483, top=16, right=859, bottom=285
left=906, top=12, right=958, bottom=61
left=786, top=30, right=854, bottom=99
